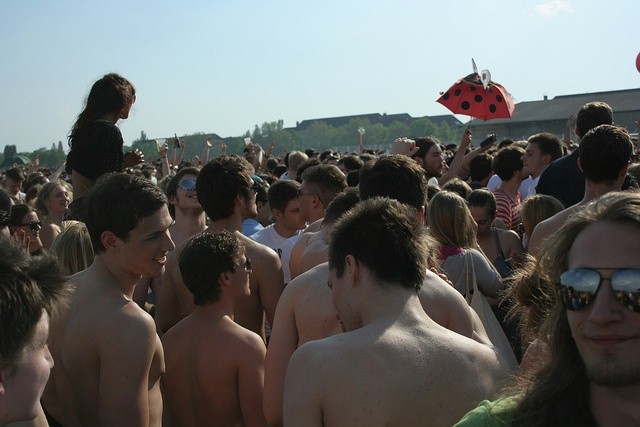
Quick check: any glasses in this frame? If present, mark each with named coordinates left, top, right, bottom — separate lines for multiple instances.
left=559, top=266, right=639, bottom=314
left=251, top=181, right=260, bottom=191
left=180, top=178, right=196, bottom=191
left=21, top=220, right=43, bottom=230
left=295, top=189, right=324, bottom=203
left=242, top=257, right=252, bottom=269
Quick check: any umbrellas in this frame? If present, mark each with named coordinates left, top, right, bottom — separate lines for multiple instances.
left=434, top=59, right=515, bottom=130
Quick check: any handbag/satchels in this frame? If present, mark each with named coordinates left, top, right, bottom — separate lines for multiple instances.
left=463, top=249, right=518, bottom=370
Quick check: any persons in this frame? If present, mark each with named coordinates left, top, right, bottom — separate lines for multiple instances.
left=518, top=194, right=564, bottom=250
left=282, top=196, right=515, bottom=427
left=288, top=189, right=361, bottom=279
left=425, top=189, right=506, bottom=301
left=466, top=188, right=525, bottom=278
left=536, top=101, right=614, bottom=209
left=32, top=180, right=73, bottom=250
left=298, top=164, right=347, bottom=223
left=123, top=132, right=289, bottom=185
left=160, top=231, right=267, bottom=427
left=451, top=192, right=640, bottom=427
left=520, top=132, right=563, bottom=201
left=238, top=181, right=273, bottom=238
left=491, top=146, right=528, bottom=268
left=48, top=220, right=93, bottom=280
left=560, top=137, right=580, bottom=156
left=421, top=127, right=529, bottom=188
left=42, top=172, right=177, bottom=427
left=0, top=154, right=73, bottom=195
left=0, top=236, right=76, bottom=427
left=289, top=136, right=420, bottom=188
left=65, top=72, right=136, bottom=200
left=625, top=120, right=640, bottom=192
left=528, top=125, right=633, bottom=261
left=158, top=156, right=284, bottom=345
left=262, top=154, right=474, bottom=427
left=8, top=205, right=43, bottom=255
left=248, top=179, right=309, bottom=285
